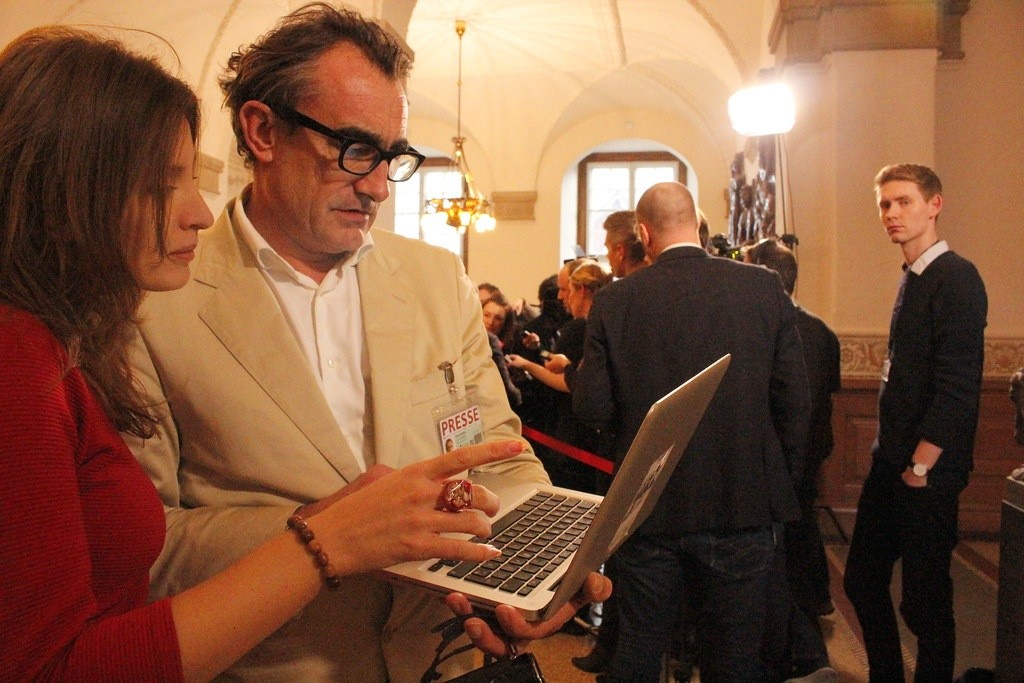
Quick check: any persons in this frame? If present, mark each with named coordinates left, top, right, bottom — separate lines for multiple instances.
left=843, top=163, right=988, bottom=683
left=0, top=9, right=613, bottom=683
left=445, top=438, right=454, bottom=452
left=478, top=181, right=842, bottom=683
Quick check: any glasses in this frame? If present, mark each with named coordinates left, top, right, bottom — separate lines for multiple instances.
left=268, top=96, right=427, bottom=184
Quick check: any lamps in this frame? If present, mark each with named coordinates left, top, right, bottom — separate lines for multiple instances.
left=421, top=19, right=497, bottom=237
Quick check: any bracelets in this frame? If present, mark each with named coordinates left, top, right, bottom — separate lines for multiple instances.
left=286, top=515, right=343, bottom=592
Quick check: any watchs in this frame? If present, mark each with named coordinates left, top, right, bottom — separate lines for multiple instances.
left=909, top=459, right=932, bottom=478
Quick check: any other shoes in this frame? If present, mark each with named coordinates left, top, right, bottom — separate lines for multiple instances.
left=571, top=639, right=617, bottom=673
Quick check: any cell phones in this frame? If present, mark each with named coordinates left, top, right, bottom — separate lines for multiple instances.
left=520, top=330, right=530, bottom=338
left=541, top=350, right=552, bottom=359
left=504, top=354, right=512, bottom=364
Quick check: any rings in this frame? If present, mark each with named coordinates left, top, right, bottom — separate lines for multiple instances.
left=442, top=479, right=473, bottom=514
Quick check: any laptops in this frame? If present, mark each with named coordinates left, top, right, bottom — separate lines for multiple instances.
left=371, top=353, right=732, bottom=623
